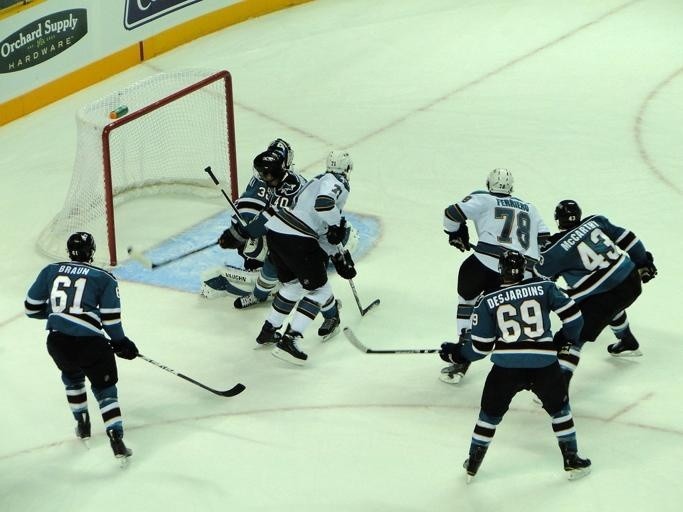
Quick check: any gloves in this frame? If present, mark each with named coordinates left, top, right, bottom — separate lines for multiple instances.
left=326, top=217, right=346, bottom=245
left=449, top=223, right=471, bottom=252
left=220, top=223, right=249, bottom=249
left=439, top=342, right=459, bottom=365
left=330, top=249, right=357, bottom=278
left=114, top=337, right=138, bottom=360
left=637, top=251, right=657, bottom=283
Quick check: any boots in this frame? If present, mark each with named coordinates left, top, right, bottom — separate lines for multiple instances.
left=463, top=446, right=488, bottom=476
left=440, top=361, right=468, bottom=377
left=78, top=411, right=91, bottom=437
left=559, top=441, right=591, bottom=470
left=234, top=294, right=258, bottom=308
left=256, top=300, right=340, bottom=360
left=108, top=429, right=133, bottom=458
left=607, top=326, right=639, bottom=353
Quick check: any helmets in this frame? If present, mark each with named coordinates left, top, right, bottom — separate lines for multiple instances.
left=327, top=150, right=352, bottom=181
left=67, top=232, right=96, bottom=261
left=267, top=137, right=294, bottom=171
left=487, top=167, right=513, bottom=196
left=553, top=199, right=582, bottom=229
left=498, top=249, right=527, bottom=284
left=254, top=150, right=284, bottom=183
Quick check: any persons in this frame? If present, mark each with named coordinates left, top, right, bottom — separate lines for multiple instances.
left=535, top=200, right=657, bottom=408
left=436, top=250, right=600, bottom=482
left=442, top=169, right=550, bottom=385
left=214, top=138, right=359, bottom=366
left=22, top=233, right=142, bottom=461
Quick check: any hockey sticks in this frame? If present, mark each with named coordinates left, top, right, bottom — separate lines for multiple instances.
left=337, top=241, right=380, bottom=316
left=102, top=336, right=245, bottom=397
left=124, top=243, right=218, bottom=269
left=342, top=326, right=439, bottom=354
